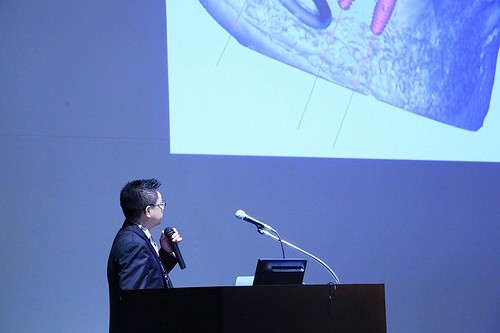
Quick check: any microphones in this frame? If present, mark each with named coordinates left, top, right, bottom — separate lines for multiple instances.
left=234, top=210, right=276, bottom=232
left=164, top=227, right=186, bottom=270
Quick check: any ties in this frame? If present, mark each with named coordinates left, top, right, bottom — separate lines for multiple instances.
left=149, top=236, right=170, bottom=288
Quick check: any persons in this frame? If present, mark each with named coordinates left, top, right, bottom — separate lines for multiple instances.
left=107, top=178, right=182, bottom=333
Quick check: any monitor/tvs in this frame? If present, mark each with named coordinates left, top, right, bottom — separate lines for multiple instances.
left=253, top=258, right=307, bottom=286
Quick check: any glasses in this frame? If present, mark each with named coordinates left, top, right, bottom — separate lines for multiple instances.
left=143, top=199, right=166, bottom=211
